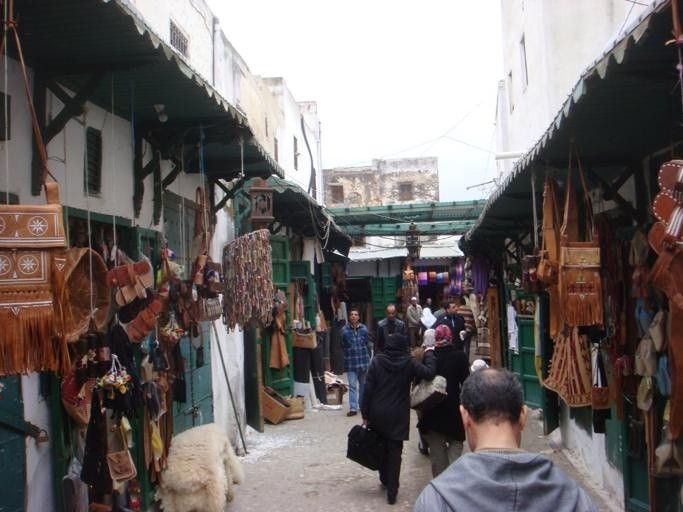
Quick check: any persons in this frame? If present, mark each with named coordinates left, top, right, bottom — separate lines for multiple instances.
left=471, top=359, right=489, bottom=372
left=361, top=334, right=436, bottom=505
left=338, top=308, right=370, bottom=416
left=374, top=297, right=476, bottom=479
left=413, top=364, right=594, bottom=512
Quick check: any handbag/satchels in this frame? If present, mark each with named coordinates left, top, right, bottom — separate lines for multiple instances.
left=558, top=237, right=601, bottom=310
left=0, top=203, right=70, bottom=376
left=86, top=411, right=106, bottom=450
left=536, top=259, right=559, bottom=284
left=410, top=373, right=447, bottom=413
left=346, top=425, right=379, bottom=470
left=107, top=451, right=138, bottom=497
left=198, top=295, right=221, bottom=322
left=80, top=451, right=105, bottom=486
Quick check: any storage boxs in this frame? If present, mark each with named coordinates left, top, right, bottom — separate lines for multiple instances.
left=325, top=386, right=343, bottom=405
left=262, top=386, right=293, bottom=425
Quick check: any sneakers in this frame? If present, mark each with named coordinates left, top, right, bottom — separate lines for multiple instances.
left=347, top=411, right=356, bottom=416
left=419, top=442, right=429, bottom=455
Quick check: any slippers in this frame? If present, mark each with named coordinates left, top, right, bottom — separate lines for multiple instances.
left=106, top=262, right=162, bottom=354
left=629, top=230, right=649, bottom=266
left=634, top=338, right=657, bottom=377
left=648, top=311, right=668, bottom=353
left=120, top=416, right=133, bottom=448
left=646, top=161, right=683, bottom=310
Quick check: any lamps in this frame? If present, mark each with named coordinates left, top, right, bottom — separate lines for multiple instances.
left=153, top=104, right=168, bottom=123
